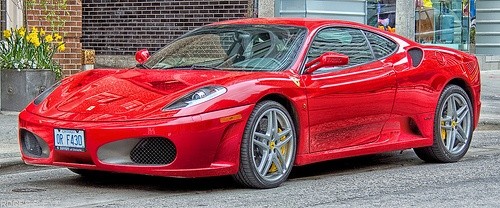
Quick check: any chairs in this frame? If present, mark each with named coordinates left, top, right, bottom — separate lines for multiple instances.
left=209, top=31, right=378, bottom=72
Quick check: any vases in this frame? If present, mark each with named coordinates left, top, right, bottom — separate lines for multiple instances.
left=0, top=68, right=56, bottom=111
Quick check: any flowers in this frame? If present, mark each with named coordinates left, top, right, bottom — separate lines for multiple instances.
left=0, top=28, right=65, bottom=78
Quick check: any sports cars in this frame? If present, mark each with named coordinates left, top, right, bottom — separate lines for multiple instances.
left=16, top=16, right=481, bottom=190
